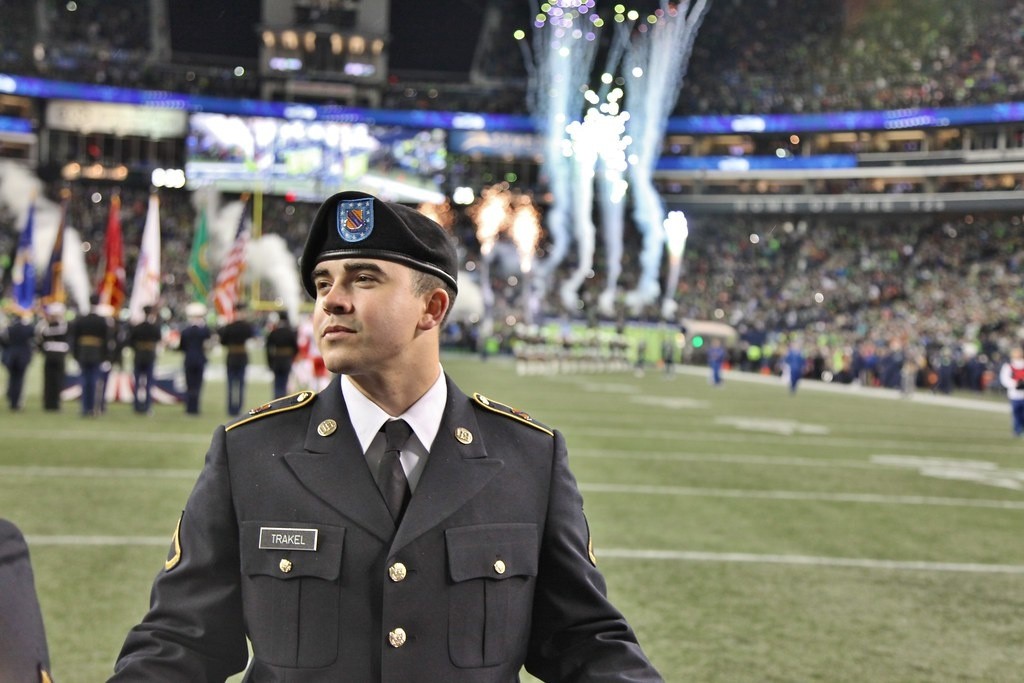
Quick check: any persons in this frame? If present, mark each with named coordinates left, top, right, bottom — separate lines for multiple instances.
left=0, top=1, right=1024, bottom=430
left=104, top=191, right=665, bottom=683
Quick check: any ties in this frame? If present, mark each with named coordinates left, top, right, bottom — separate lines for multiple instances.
left=377, top=420, right=411, bottom=530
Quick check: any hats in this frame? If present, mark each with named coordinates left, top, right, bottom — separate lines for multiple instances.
left=301, top=191, right=458, bottom=300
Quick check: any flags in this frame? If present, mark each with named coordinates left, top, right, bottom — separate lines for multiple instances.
left=2, top=204, right=37, bottom=322
left=217, top=224, right=244, bottom=317
left=185, top=208, right=212, bottom=321
left=95, top=199, right=123, bottom=315
left=41, top=216, right=69, bottom=317
left=132, top=195, right=161, bottom=323
